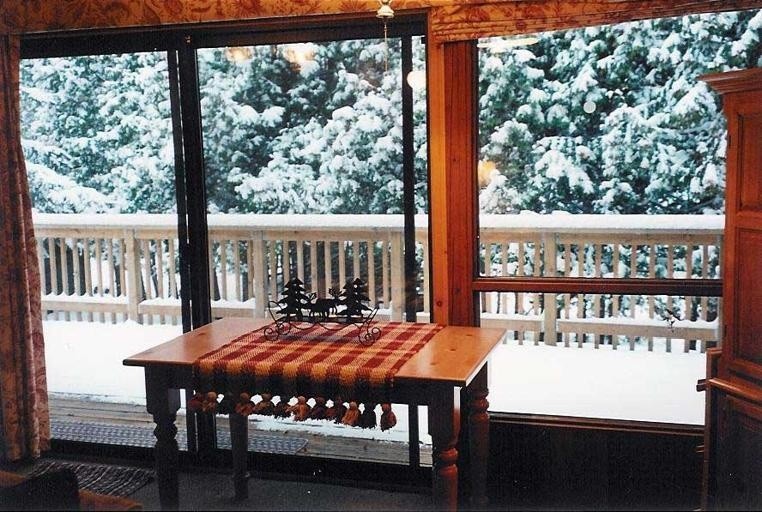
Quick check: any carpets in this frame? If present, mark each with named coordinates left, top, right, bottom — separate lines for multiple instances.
left=23, top=458, right=155, bottom=498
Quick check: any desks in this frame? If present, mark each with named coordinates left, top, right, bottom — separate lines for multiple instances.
left=123, top=299, right=506, bottom=512
